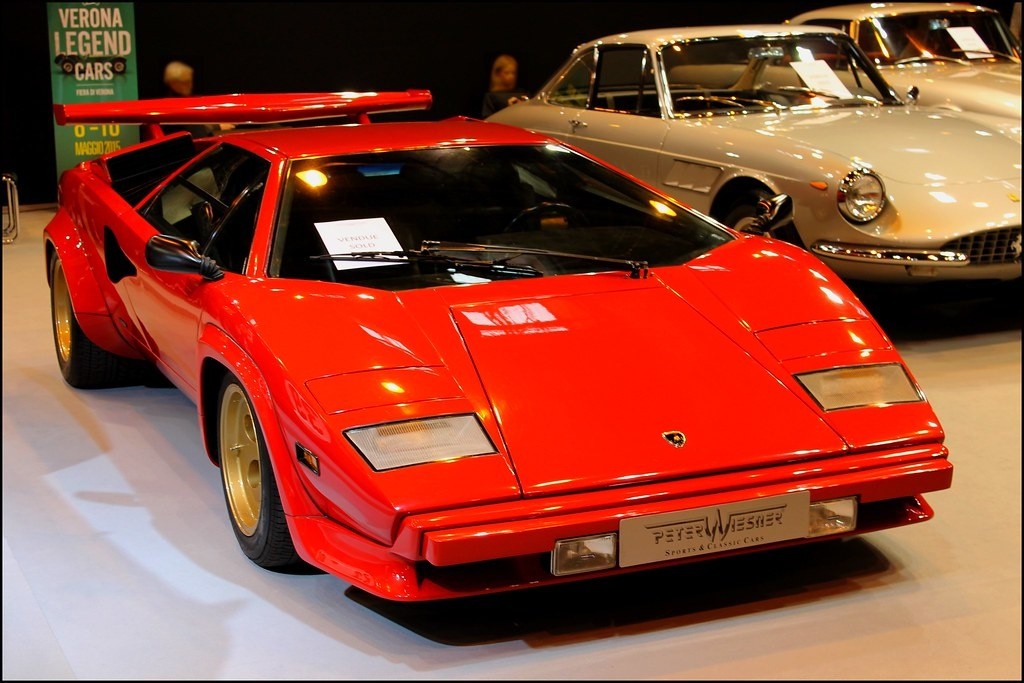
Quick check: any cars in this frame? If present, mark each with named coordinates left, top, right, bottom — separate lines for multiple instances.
left=670, top=4, right=1022, bottom=122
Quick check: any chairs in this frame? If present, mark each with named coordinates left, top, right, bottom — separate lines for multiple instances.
left=416, top=158, right=543, bottom=273
left=284, top=168, right=378, bottom=279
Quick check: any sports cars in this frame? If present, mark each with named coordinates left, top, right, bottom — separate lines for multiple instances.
left=42, top=90, right=953, bottom=604
left=484, top=24, right=1023, bottom=285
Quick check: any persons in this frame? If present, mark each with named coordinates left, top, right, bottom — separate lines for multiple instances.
left=483, top=56, right=529, bottom=116
left=157, top=62, right=197, bottom=135
